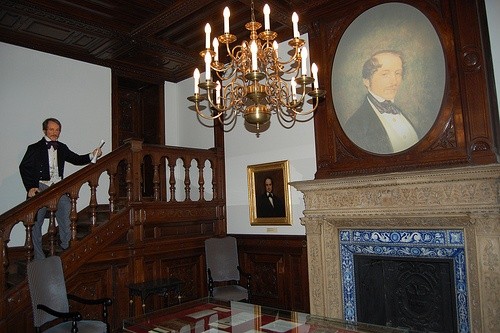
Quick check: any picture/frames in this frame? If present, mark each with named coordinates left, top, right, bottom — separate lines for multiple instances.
left=246, top=160, right=293, bottom=226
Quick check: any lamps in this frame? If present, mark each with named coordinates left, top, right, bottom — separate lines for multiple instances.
left=187, top=0, right=327, bottom=130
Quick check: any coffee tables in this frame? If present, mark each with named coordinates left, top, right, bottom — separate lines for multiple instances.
left=126, top=278, right=186, bottom=317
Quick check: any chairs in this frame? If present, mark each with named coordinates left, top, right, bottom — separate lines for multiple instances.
left=205, top=237, right=252, bottom=303
left=27, top=255, right=113, bottom=333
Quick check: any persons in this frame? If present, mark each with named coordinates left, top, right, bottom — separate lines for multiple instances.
left=19, top=118, right=102, bottom=258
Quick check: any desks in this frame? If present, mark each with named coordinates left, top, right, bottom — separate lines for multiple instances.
left=123, top=296, right=409, bottom=333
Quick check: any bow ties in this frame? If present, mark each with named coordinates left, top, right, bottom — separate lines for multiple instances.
left=44, top=138, right=58, bottom=150
left=268, top=193, right=273, bottom=198
